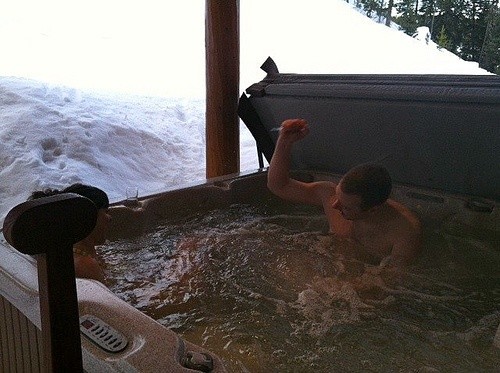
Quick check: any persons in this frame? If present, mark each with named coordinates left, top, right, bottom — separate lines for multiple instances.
left=31, top=181, right=112, bottom=287
left=263, top=119, right=423, bottom=278
